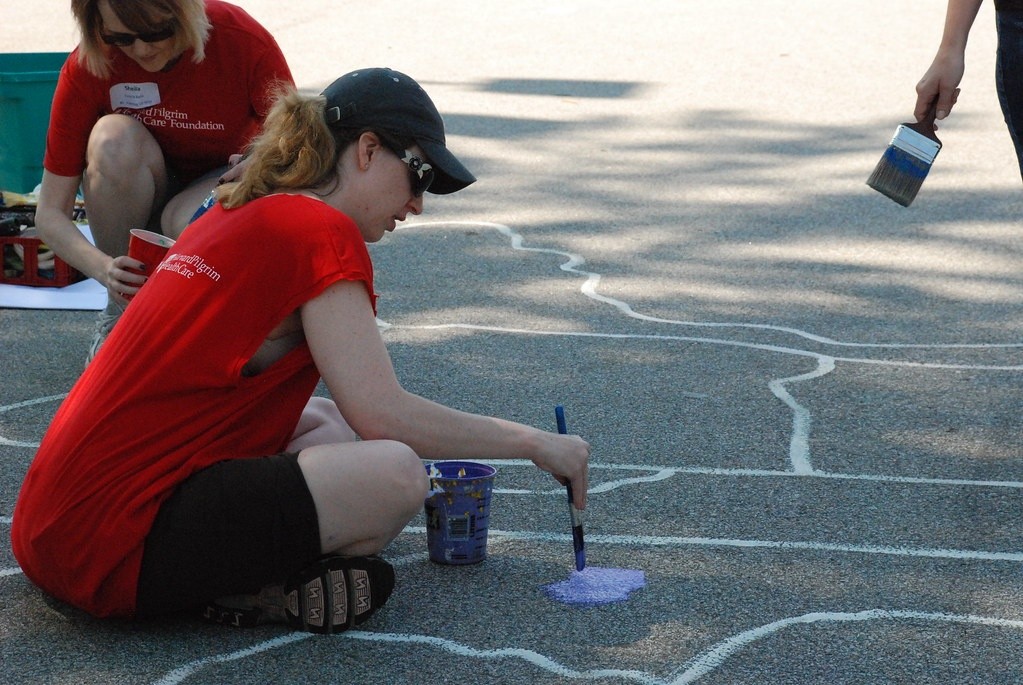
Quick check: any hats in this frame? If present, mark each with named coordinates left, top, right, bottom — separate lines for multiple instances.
left=320, top=68, right=475, bottom=195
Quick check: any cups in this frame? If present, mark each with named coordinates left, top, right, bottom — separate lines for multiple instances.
left=118, top=228, right=176, bottom=302
left=424, top=461, right=497, bottom=565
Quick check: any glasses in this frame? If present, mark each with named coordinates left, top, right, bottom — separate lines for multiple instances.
left=98, top=20, right=178, bottom=46
left=374, top=126, right=437, bottom=198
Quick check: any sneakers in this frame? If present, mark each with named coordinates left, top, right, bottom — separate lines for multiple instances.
left=84, top=301, right=128, bottom=373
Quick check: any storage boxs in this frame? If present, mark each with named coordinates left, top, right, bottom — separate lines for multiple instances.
left=0, top=52, right=84, bottom=195
left=0, top=236, right=90, bottom=288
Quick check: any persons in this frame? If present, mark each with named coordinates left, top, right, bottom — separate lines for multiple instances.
left=34, top=0, right=298, bottom=368
left=913, top=0, right=1023, bottom=181
left=11, top=67, right=590, bottom=636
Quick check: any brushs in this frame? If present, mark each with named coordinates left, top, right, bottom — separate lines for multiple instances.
left=864, top=94, right=942, bottom=208
left=555, top=406, right=585, bottom=572
left=178, top=182, right=219, bottom=240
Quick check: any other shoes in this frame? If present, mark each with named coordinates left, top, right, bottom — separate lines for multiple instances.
left=191, top=554, right=396, bottom=636
